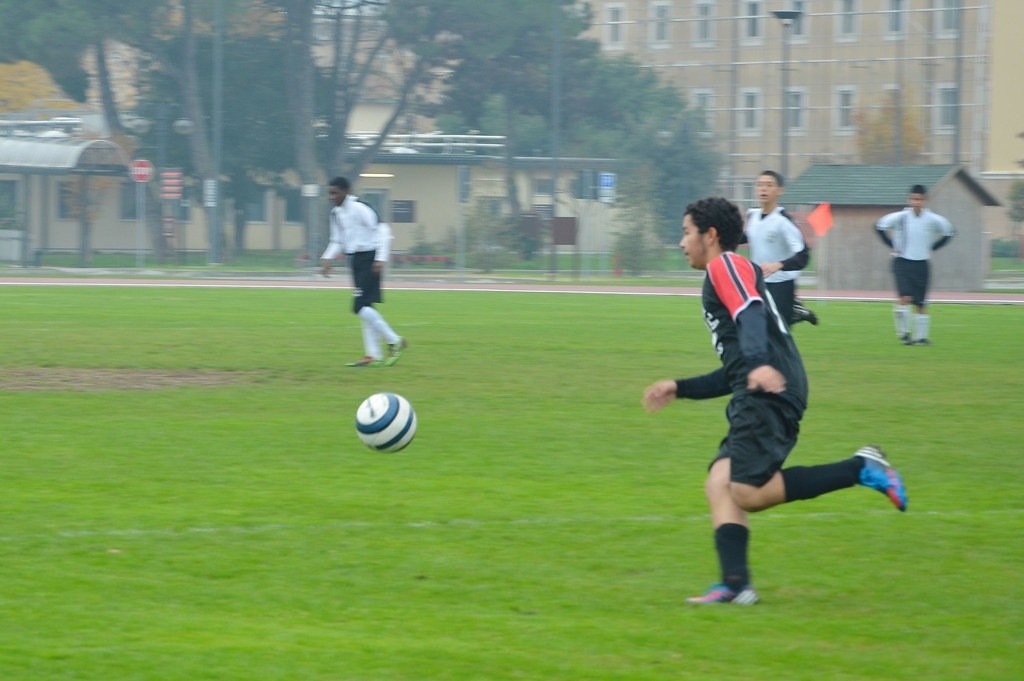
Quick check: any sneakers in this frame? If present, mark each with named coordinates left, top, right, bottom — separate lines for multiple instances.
left=386, top=337, right=407, bottom=366
left=345, top=355, right=385, bottom=369
left=854, top=444, right=907, bottom=513
left=793, top=299, right=816, bottom=325
left=687, top=582, right=759, bottom=605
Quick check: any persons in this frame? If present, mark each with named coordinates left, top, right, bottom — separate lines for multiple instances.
left=644, top=197, right=907, bottom=609
left=873, top=185, right=954, bottom=346
left=319, top=177, right=406, bottom=366
left=734, top=170, right=817, bottom=329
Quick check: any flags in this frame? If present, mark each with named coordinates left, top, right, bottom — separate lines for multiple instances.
left=806, top=203, right=833, bottom=235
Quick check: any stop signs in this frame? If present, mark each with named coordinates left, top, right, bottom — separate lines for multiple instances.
left=128, top=159, right=155, bottom=183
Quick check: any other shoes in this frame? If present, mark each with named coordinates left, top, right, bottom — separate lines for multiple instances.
left=901, top=331, right=928, bottom=347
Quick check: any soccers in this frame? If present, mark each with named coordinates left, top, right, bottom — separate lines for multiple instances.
left=354, top=393, right=420, bottom=455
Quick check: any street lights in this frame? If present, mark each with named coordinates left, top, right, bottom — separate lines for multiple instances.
left=770, top=8, right=804, bottom=187
